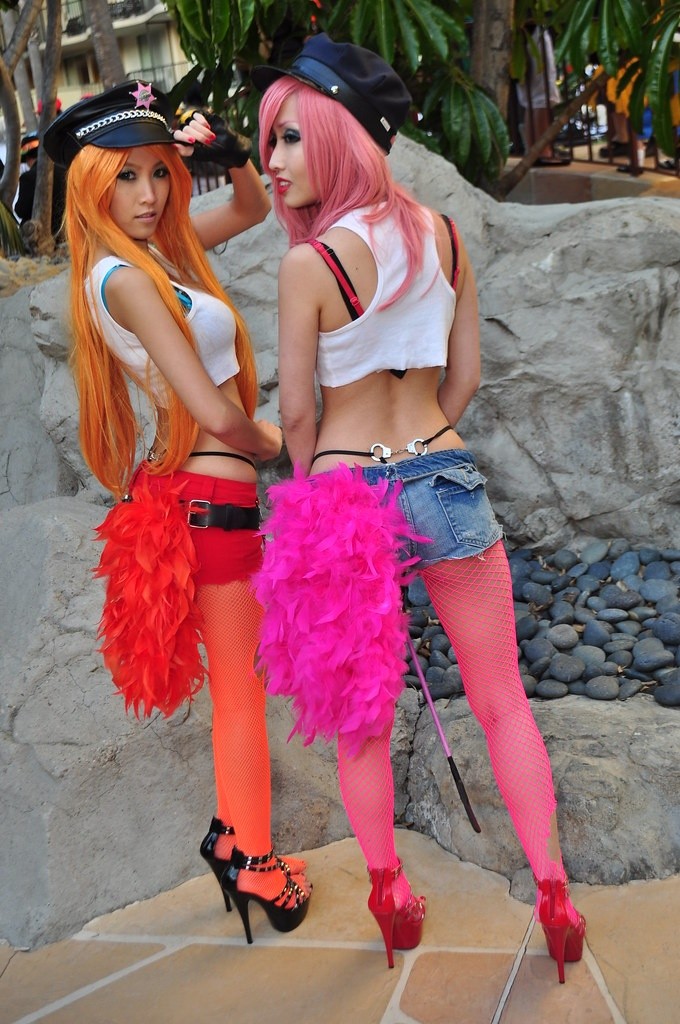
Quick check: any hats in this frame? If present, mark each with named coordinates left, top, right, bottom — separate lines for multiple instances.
left=42, top=80, right=192, bottom=166
left=249, top=32, right=412, bottom=155
left=19, top=131, right=40, bottom=164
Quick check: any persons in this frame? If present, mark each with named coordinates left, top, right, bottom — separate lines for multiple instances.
left=0, top=96, right=70, bottom=252
left=515, top=9, right=680, bottom=176
left=257, top=38, right=585, bottom=983
left=42, top=80, right=314, bottom=944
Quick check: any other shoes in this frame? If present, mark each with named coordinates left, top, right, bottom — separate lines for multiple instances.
left=531, top=157, right=572, bottom=168
left=616, top=165, right=643, bottom=175
left=655, top=160, right=676, bottom=172
left=600, top=140, right=632, bottom=157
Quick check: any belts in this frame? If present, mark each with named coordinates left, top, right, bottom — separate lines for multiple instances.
left=178, top=498, right=261, bottom=531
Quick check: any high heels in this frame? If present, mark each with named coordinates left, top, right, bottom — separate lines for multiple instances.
left=368, top=855, right=425, bottom=968
left=220, top=846, right=312, bottom=944
left=532, top=871, right=586, bottom=984
left=202, top=816, right=292, bottom=911
